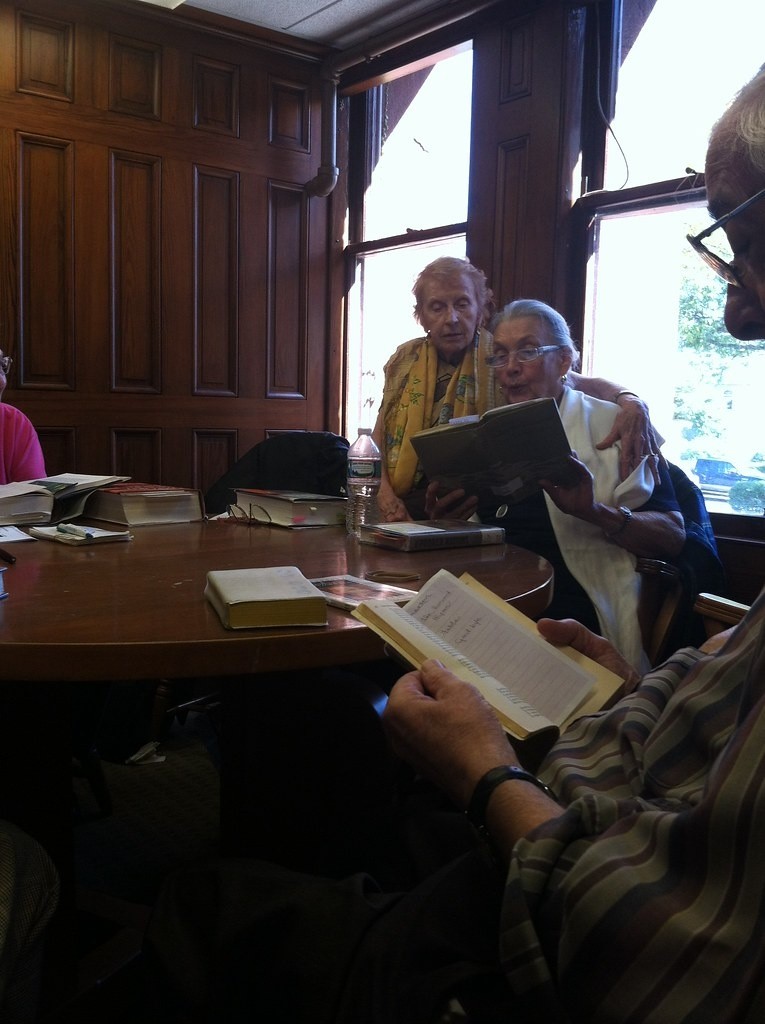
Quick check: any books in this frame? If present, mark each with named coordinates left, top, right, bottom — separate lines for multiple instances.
left=203, top=565, right=329, bottom=629
left=307, top=574, right=418, bottom=612
left=0, top=472, right=208, bottom=546
left=409, top=397, right=580, bottom=515
left=227, top=487, right=348, bottom=528
left=349, top=568, right=626, bottom=741
left=357, top=516, right=506, bottom=552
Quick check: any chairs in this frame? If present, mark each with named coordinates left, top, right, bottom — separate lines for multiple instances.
left=203, top=432, right=349, bottom=513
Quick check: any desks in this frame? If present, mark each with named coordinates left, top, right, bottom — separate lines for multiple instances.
left=0, top=494, right=554, bottom=869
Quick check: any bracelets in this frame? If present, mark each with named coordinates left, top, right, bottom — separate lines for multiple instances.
left=601, top=505, right=632, bottom=538
left=464, top=764, right=560, bottom=837
left=613, top=390, right=637, bottom=404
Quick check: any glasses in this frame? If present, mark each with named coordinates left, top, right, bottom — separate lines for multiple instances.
left=485, top=344, right=565, bottom=368
left=686, top=189, right=765, bottom=288
left=0, top=356, right=12, bottom=374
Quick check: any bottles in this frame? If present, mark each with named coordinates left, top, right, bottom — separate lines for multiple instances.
left=346, top=428, right=381, bottom=538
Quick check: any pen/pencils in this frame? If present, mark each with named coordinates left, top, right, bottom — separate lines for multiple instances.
left=57, top=522, right=94, bottom=539
left=0, top=548, right=17, bottom=564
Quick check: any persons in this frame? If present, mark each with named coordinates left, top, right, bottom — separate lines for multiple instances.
left=370, top=256, right=670, bottom=524
left=0, top=348, right=47, bottom=485
left=378, top=60, right=764, bottom=1024
left=423, top=299, right=687, bottom=637
